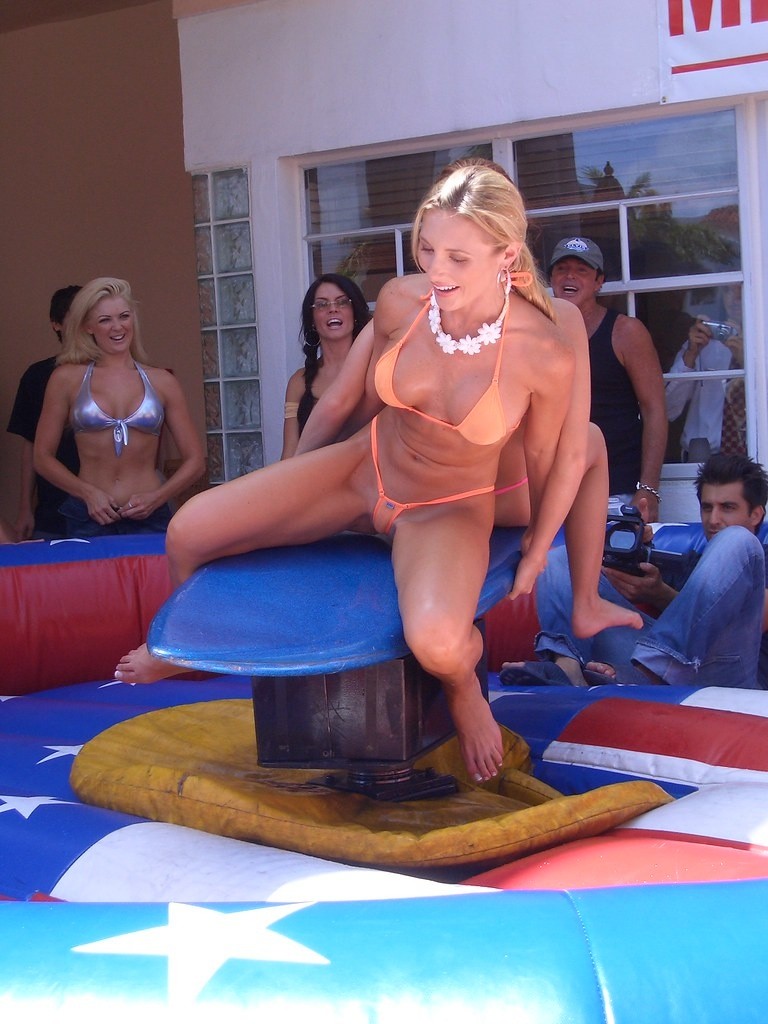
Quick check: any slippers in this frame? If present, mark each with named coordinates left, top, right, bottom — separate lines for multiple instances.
left=500, top=660, right=573, bottom=687
left=583, top=660, right=652, bottom=686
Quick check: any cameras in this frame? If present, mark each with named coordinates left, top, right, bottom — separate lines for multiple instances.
left=702, top=320, right=733, bottom=340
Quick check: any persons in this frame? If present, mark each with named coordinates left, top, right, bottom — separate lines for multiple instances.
left=281, top=273, right=370, bottom=463
left=30, top=278, right=207, bottom=537
left=663, top=266, right=750, bottom=462
left=114, top=168, right=576, bottom=783
left=291, top=156, right=646, bottom=639
left=544, top=234, right=667, bottom=522
left=4, top=286, right=81, bottom=544
left=493, top=452, right=767, bottom=685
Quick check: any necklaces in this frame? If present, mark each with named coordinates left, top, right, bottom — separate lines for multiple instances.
left=426, top=288, right=505, bottom=354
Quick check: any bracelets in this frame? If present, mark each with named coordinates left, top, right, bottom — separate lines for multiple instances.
left=637, top=481, right=661, bottom=502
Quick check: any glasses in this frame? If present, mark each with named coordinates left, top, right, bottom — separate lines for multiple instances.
left=310, top=297, right=352, bottom=311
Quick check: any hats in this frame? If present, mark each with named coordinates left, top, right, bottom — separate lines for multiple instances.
left=550, top=237, right=603, bottom=273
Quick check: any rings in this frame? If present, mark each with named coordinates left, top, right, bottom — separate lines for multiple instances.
left=127, top=502, right=133, bottom=508
